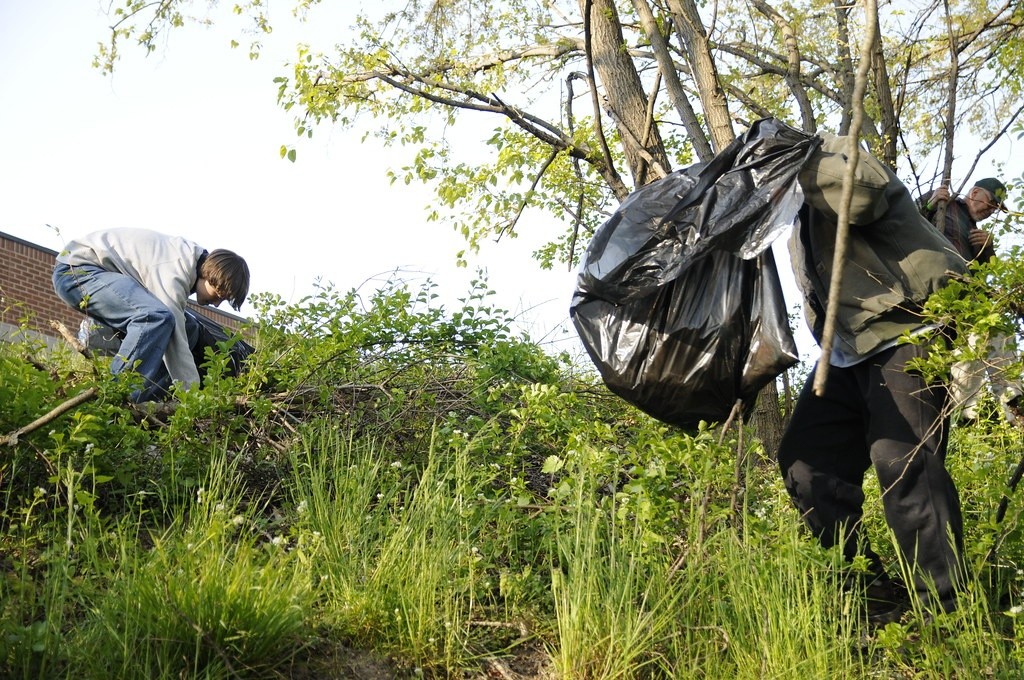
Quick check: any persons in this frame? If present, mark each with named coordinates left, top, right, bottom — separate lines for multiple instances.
left=751, top=118, right=972, bottom=659
left=914, top=178, right=1024, bottom=429
left=52, top=226, right=250, bottom=444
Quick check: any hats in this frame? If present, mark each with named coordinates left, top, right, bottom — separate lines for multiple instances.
left=974, top=177, right=1010, bottom=214
